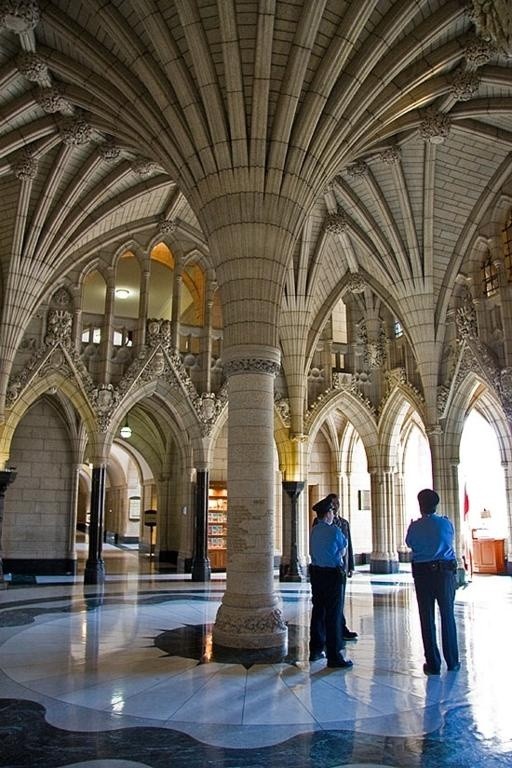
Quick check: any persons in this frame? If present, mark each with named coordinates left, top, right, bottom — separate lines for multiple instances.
left=309, top=500, right=353, bottom=667
left=422, top=671, right=463, bottom=768
left=311, top=494, right=358, bottom=638
left=405, top=489, right=461, bottom=675
left=311, top=668, right=356, bottom=767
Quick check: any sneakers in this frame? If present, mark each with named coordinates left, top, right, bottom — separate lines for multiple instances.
left=342, top=626, right=358, bottom=639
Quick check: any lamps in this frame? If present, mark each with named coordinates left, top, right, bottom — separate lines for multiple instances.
left=120, top=412, right=132, bottom=440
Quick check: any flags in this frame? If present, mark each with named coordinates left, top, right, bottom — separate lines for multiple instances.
left=463, top=487, right=469, bottom=522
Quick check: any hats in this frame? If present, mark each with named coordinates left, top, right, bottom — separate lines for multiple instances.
left=312, top=497, right=334, bottom=512
left=418, top=489, right=440, bottom=505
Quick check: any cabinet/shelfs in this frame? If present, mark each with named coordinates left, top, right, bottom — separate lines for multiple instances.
left=471, top=539, right=506, bottom=574
left=206, top=480, right=228, bottom=569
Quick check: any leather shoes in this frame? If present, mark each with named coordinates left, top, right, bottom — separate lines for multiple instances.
left=309, top=654, right=353, bottom=668
left=423, top=662, right=461, bottom=676
